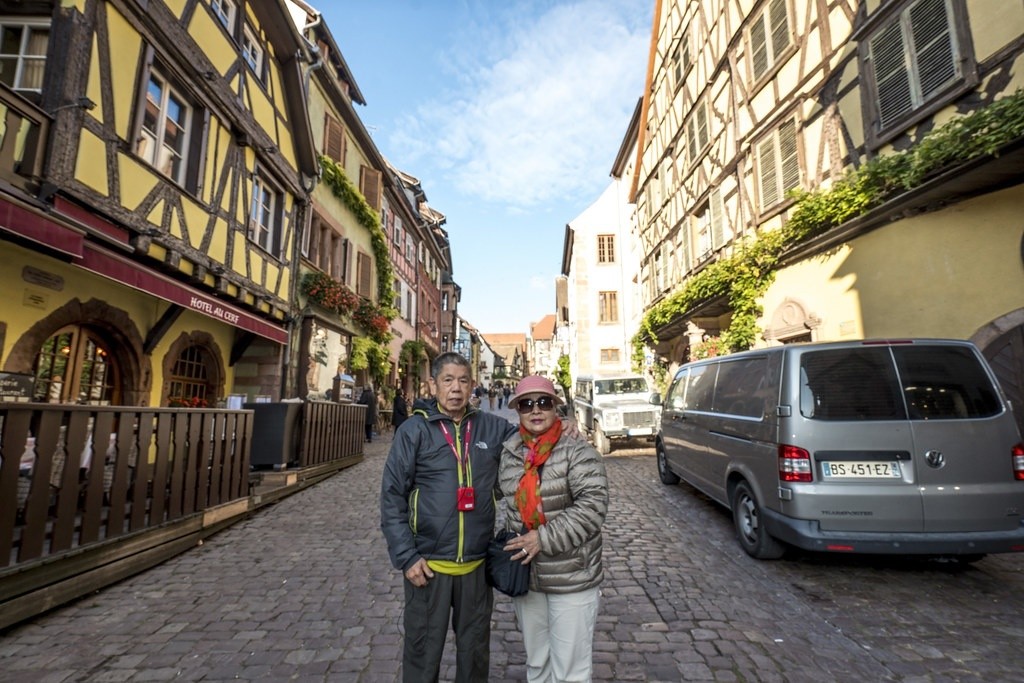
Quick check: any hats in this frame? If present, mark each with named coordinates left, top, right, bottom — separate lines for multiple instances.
left=507, top=375, right=564, bottom=408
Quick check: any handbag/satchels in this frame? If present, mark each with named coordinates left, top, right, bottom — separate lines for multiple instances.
left=486, top=530, right=530, bottom=596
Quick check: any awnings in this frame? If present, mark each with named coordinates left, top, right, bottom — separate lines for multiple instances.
left=0, top=189, right=87, bottom=259
left=46, top=235, right=292, bottom=349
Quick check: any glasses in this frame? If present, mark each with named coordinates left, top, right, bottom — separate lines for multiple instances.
left=517, top=396, right=553, bottom=413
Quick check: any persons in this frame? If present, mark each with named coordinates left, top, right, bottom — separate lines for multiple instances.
left=354, top=380, right=377, bottom=445
left=494, top=375, right=610, bottom=683
left=378, top=348, right=583, bottom=683
left=391, top=387, right=411, bottom=443
left=470, top=380, right=512, bottom=413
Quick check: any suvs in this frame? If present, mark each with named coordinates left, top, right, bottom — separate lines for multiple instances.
left=573, top=373, right=665, bottom=456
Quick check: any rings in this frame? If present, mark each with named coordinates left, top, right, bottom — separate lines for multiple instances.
left=522, top=548, right=529, bottom=556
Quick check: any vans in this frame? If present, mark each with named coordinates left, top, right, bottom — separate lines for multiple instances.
left=552, top=385, right=568, bottom=416
left=647, top=335, right=1024, bottom=570
